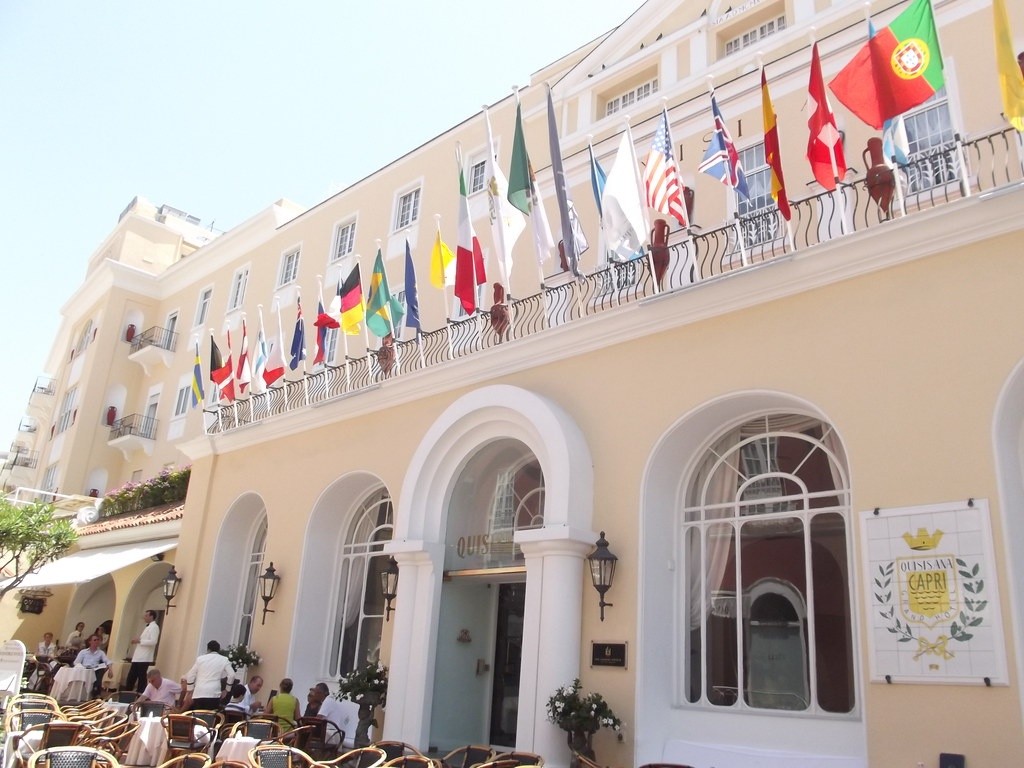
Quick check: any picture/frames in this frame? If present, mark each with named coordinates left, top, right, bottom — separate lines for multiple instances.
left=856, top=497, right=1010, bottom=691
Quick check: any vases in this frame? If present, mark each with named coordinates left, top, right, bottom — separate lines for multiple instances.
left=565, top=729, right=597, bottom=768
left=349, top=691, right=384, bottom=748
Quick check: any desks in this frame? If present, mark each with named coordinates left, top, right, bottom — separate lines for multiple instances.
left=3, top=730, right=45, bottom=768
left=215, top=736, right=278, bottom=768
left=49, top=667, right=97, bottom=702
left=124, top=717, right=214, bottom=767
left=101, top=701, right=135, bottom=715
left=101, top=663, right=132, bottom=693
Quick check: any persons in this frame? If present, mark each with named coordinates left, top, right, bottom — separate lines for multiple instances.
left=126, top=610, right=160, bottom=693
left=304, top=687, right=322, bottom=716
left=97, top=627, right=109, bottom=650
left=225, top=675, right=263, bottom=719
left=39, top=632, right=57, bottom=661
left=264, top=678, right=301, bottom=734
left=65, top=622, right=85, bottom=651
left=136, top=668, right=185, bottom=718
left=315, top=683, right=346, bottom=744
left=73, top=635, right=113, bottom=698
left=186, top=640, right=236, bottom=710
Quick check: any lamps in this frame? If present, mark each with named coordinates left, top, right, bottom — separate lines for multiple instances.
left=258, top=561, right=281, bottom=627
left=161, top=565, right=182, bottom=616
left=585, top=529, right=619, bottom=624
left=376, top=555, right=400, bottom=624
left=151, top=553, right=164, bottom=562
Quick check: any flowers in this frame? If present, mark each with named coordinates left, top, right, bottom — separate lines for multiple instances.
left=98, top=463, right=194, bottom=518
left=545, top=676, right=622, bottom=735
left=220, top=641, right=263, bottom=671
left=328, top=657, right=388, bottom=706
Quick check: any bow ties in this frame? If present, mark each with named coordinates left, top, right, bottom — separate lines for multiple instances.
left=147, top=624, right=149, bottom=626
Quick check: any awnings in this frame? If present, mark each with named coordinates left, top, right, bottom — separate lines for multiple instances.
left=0, top=537, right=178, bottom=590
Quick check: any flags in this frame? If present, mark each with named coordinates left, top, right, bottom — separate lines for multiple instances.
left=806, top=41, right=847, bottom=190
left=643, top=107, right=687, bottom=228
left=589, top=145, right=645, bottom=261
left=761, top=66, right=791, bottom=221
left=547, top=91, right=590, bottom=284
left=191, top=91, right=591, bottom=410
left=698, top=87, right=750, bottom=204
left=868, top=19, right=911, bottom=165
left=993, top=0, right=1024, bottom=131
left=601, top=125, right=650, bottom=262
left=828, top=0, right=945, bottom=129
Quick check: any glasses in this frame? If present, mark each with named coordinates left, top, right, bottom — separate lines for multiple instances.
left=308, top=694, right=315, bottom=698
left=93, top=639, right=100, bottom=642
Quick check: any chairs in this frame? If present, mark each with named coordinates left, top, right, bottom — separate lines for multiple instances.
left=1, top=649, right=696, bottom=768
